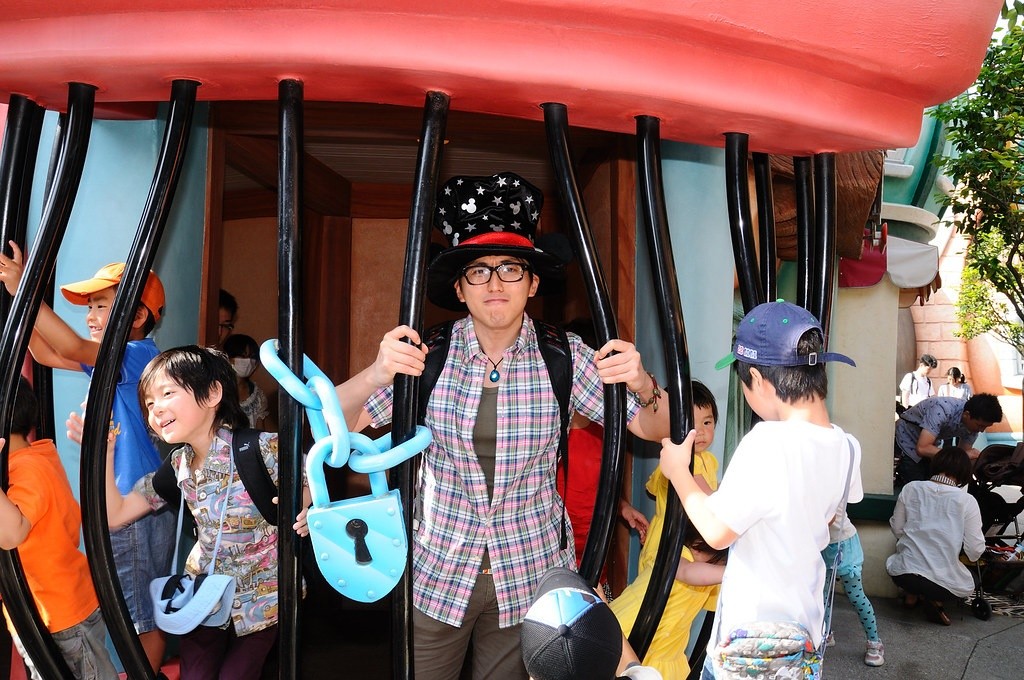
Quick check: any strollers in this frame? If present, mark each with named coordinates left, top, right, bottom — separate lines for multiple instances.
left=962, top=443, right=1023, bottom=621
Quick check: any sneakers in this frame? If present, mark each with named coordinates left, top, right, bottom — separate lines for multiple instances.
left=826, top=631, right=835, bottom=646
left=865, top=638, right=884, bottom=666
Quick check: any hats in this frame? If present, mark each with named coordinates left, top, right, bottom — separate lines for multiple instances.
left=149, top=445, right=236, bottom=636
left=425, top=171, right=563, bottom=312
left=520, top=567, right=623, bottom=680
left=60, top=262, right=165, bottom=325
left=715, top=298, right=857, bottom=371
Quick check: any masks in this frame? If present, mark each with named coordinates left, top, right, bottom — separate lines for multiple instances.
left=230, top=358, right=258, bottom=377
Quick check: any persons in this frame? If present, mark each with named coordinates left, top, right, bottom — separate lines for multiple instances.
left=0, top=240, right=177, bottom=679
left=218, top=290, right=238, bottom=347
left=894, top=393, right=1003, bottom=483
left=0, top=375, right=119, bottom=680
left=521, top=318, right=727, bottom=680
left=938, top=367, right=974, bottom=448
left=820, top=433, right=885, bottom=666
left=219, top=333, right=270, bottom=430
left=900, top=355, right=938, bottom=410
left=887, top=447, right=986, bottom=624
left=332, top=171, right=672, bottom=680
left=65, top=345, right=313, bottom=679
left=660, top=297, right=854, bottom=680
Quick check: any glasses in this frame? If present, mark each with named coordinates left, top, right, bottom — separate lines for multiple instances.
left=218, top=323, right=234, bottom=330
left=460, top=263, right=532, bottom=285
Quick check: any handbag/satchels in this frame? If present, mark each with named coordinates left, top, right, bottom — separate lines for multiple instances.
left=712, top=620, right=823, bottom=680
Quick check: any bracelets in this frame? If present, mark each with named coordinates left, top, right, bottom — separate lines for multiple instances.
left=634, top=373, right=661, bottom=412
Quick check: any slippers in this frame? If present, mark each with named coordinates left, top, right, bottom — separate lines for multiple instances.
left=924, top=598, right=952, bottom=626
left=901, top=593, right=920, bottom=608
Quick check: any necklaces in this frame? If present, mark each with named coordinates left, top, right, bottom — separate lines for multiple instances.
left=930, top=475, right=956, bottom=488
left=481, top=349, right=503, bottom=382
